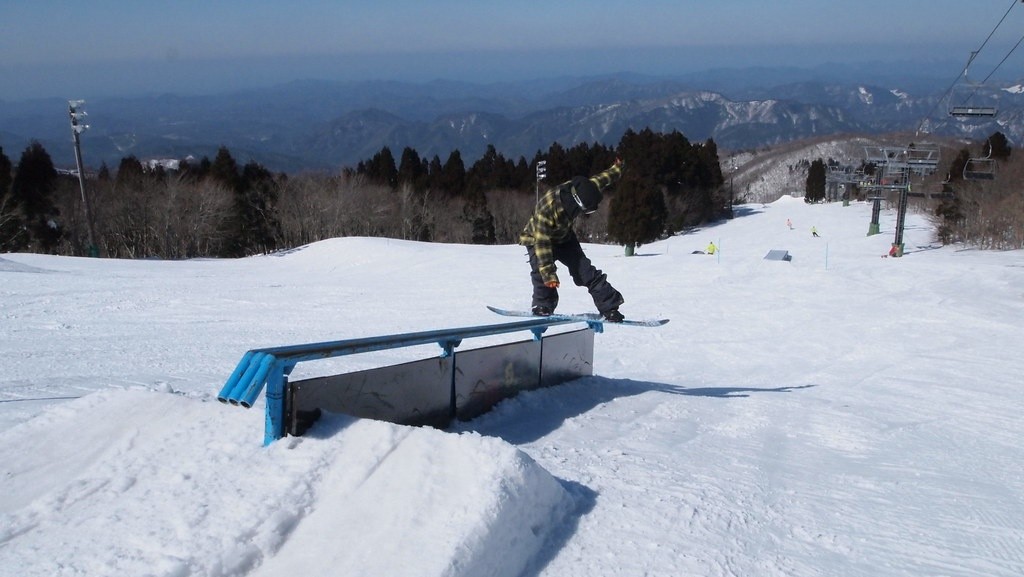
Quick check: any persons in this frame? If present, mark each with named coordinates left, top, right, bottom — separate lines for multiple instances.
left=810, top=226, right=818, bottom=237
left=705, top=241, right=719, bottom=254
left=519, top=152, right=627, bottom=322
left=785, top=219, right=792, bottom=227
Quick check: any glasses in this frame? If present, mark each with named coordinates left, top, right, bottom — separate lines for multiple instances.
left=571, top=186, right=598, bottom=217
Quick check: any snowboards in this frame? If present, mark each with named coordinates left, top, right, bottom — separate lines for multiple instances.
left=485, top=305, right=672, bottom=330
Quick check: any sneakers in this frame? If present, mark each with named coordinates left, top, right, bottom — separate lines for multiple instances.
left=532, top=305, right=553, bottom=316
left=606, top=310, right=624, bottom=322
